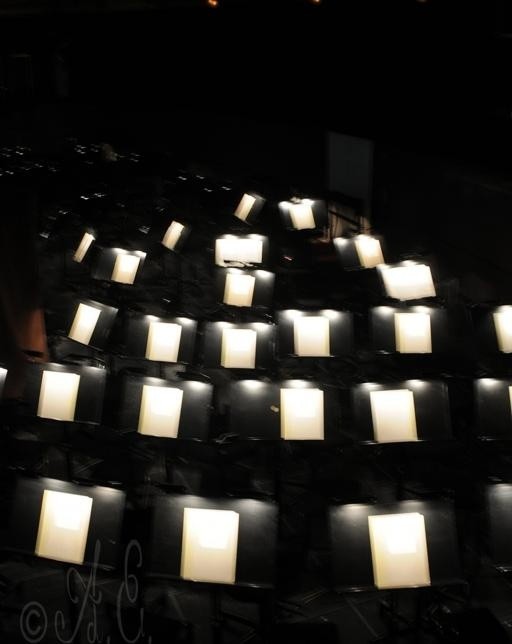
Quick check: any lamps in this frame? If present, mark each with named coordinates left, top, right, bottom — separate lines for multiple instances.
left=67, top=188, right=439, bottom=374
left=176, top=501, right=243, bottom=588
left=367, top=387, right=419, bottom=445
left=35, top=368, right=82, bottom=424
left=32, top=487, right=98, bottom=566
left=489, top=302, right=512, bottom=355
left=279, top=386, right=326, bottom=444
left=135, top=383, right=186, bottom=438
left=362, top=509, right=432, bottom=592
